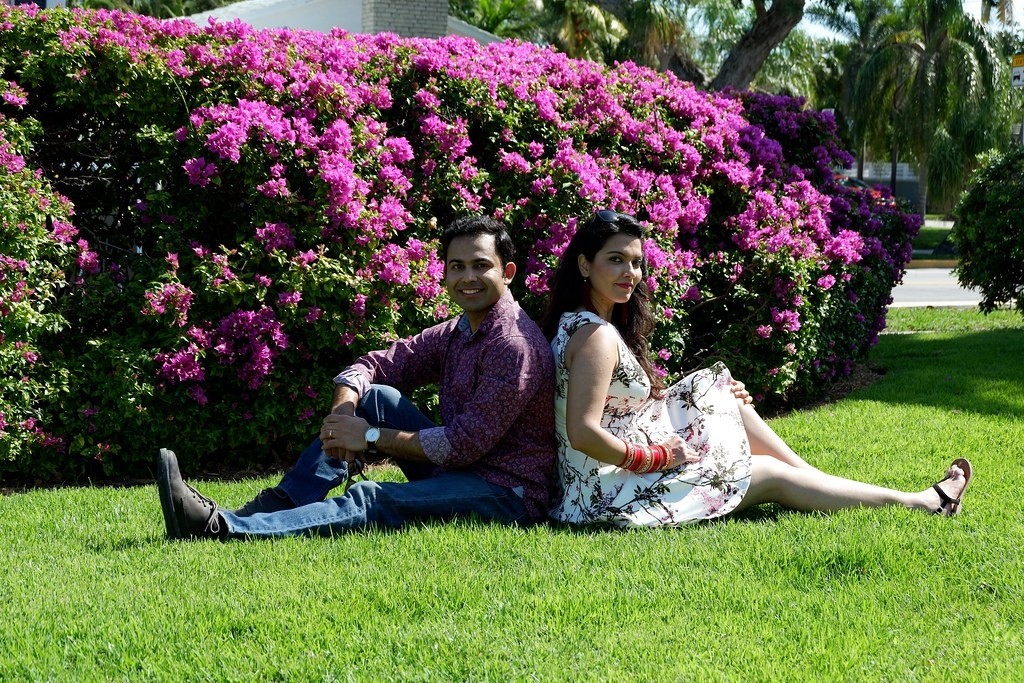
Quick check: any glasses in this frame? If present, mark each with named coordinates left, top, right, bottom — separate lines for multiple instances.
left=589, top=210, right=640, bottom=230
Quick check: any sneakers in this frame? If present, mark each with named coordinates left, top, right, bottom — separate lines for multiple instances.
left=159, top=448, right=225, bottom=541
left=233, top=486, right=296, bottom=518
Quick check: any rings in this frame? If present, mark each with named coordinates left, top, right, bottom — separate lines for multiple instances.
left=329, top=430, right=332, bottom=438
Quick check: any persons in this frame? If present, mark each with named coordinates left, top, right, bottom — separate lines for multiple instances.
left=550, top=209, right=972, bottom=529
left=155, top=216, right=562, bottom=541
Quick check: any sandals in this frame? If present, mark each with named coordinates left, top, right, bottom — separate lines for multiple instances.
left=930, top=458, right=972, bottom=516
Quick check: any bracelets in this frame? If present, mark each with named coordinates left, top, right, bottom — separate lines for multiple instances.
left=615, top=439, right=673, bottom=475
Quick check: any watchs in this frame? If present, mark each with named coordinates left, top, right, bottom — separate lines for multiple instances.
left=364, top=426, right=381, bottom=450
